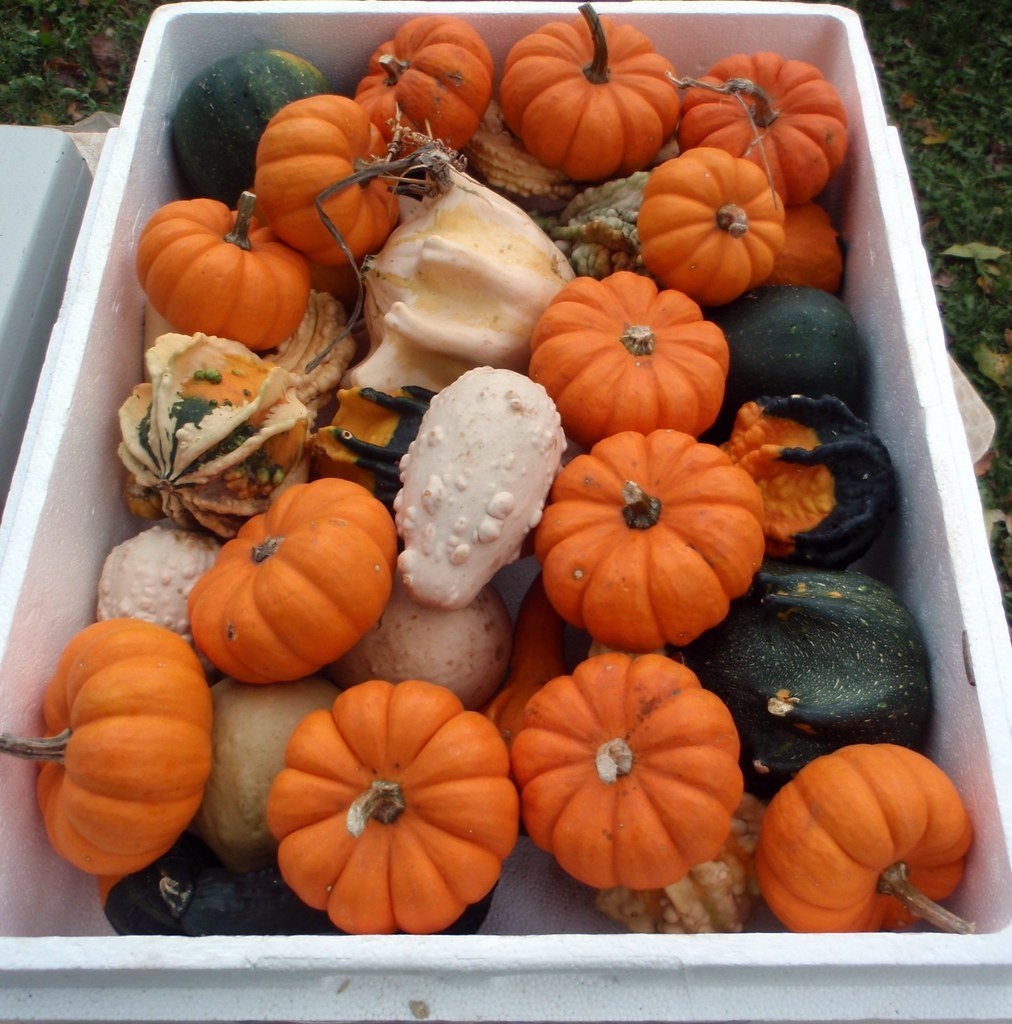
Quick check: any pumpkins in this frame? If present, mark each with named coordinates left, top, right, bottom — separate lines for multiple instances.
left=0, top=3, right=980, bottom=935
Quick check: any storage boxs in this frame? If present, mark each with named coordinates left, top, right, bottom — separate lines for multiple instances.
left=0, top=0, right=1012, bottom=1023
